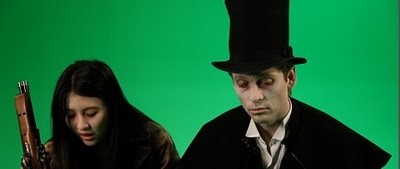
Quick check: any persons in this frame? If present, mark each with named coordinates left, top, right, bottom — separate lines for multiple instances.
left=43, top=59, right=180, bottom=169
left=176, top=44, right=392, bottom=169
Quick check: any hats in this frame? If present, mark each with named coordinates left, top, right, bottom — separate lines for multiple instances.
left=211, top=0, right=307, bottom=75
